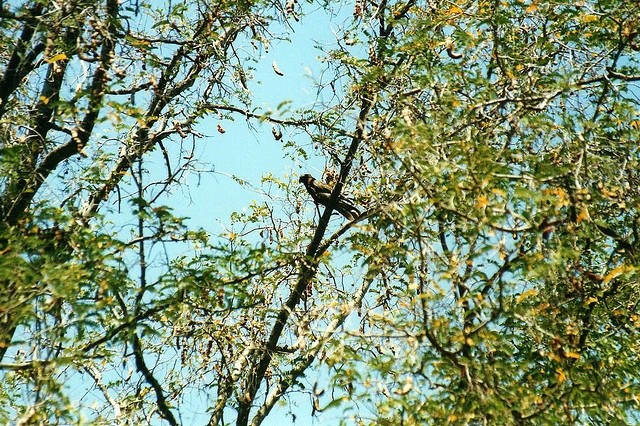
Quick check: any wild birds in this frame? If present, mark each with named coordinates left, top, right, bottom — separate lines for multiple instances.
left=299, top=174, right=361, bottom=222
